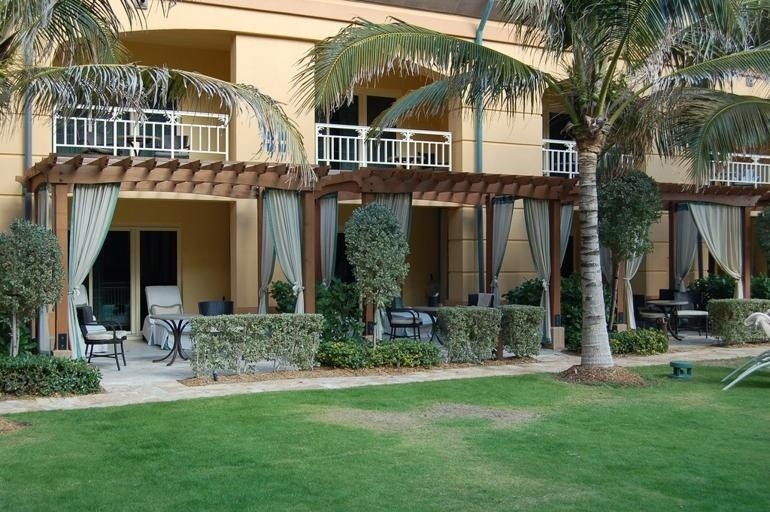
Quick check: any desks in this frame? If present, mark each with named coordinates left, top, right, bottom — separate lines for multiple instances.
left=108, top=135, right=161, bottom=156
left=150, top=314, right=203, bottom=366
left=387, top=156, right=426, bottom=170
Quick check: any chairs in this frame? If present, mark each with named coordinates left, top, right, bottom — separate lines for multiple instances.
left=721, top=312, right=770, bottom=392
left=143, top=286, right=206, bottom=349
left=154, top=135, right=190, bottom=158
left=417, top=152, right=448, bottom=172
left=77, top=129, right=122, bottom=156
left=381, top=293, right=493, bottom=343
left=76, top=306, right=127, bottom=371
left=74, top=284, right=108, bottom=358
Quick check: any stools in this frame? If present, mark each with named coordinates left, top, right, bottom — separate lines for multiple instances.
left=633, top=289, right=709, bottom=339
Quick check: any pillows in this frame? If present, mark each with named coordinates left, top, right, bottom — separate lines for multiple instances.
left=151, top=304, right=182, bottom=314
